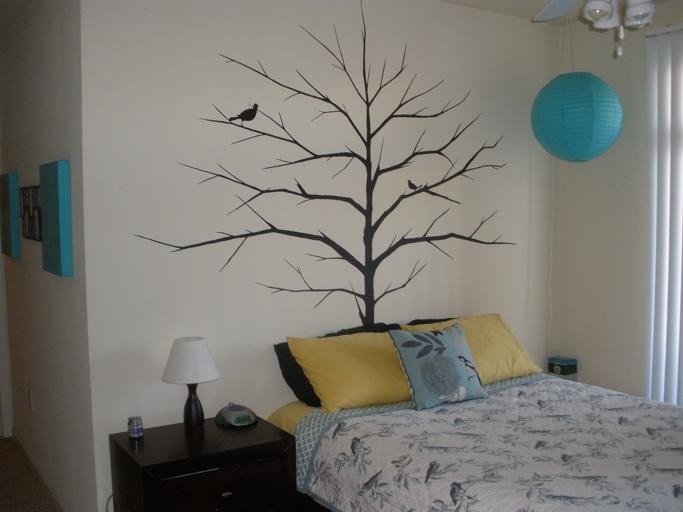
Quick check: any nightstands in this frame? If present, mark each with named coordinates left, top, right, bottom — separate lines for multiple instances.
left=109, top=417, right=297, bottom=512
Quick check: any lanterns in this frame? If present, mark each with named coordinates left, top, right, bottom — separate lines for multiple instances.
left=530, top=72, right=623, bottom=163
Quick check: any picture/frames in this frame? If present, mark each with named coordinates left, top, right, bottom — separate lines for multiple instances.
left=18, top=185, right=42, bottom=242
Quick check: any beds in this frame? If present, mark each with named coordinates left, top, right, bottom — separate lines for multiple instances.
left=270, top=373, right=682, bottom=512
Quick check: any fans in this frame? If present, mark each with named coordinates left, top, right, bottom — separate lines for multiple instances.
left=533, top=0, right=580, bottom=24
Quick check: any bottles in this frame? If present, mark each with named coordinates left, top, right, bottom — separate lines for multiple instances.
left=127, top=416, right=143, bottom=438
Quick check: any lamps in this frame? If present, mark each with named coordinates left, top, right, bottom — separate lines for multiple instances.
left=580, top=0, right=652, bottom=29
left=531, top=19, right=623, bottom=162
left=161, top=337, right=222, bottom=431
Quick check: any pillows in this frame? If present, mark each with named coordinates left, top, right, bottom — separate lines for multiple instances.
left=286, top=332, right=414, bottom=414
left=408, top=318, right=455, bottom=325
left=386, top=323, right=490, bottom=411
left=274, top=324, right=404, bottom=408
left=399, top=313, right=541, bottom=387
